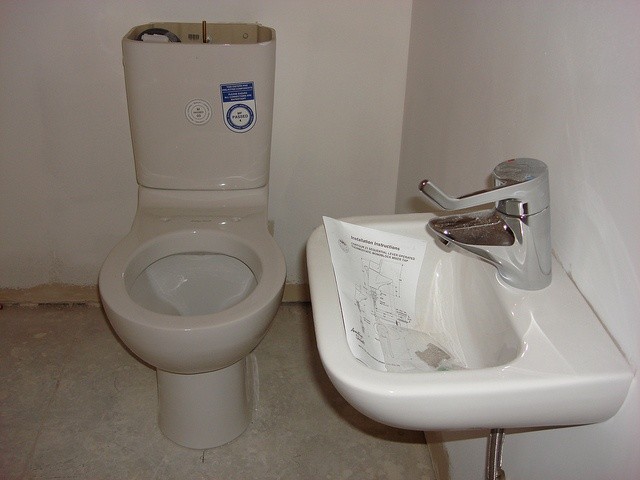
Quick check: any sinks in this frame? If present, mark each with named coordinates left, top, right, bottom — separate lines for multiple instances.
left=306, top=212, right=632, bottom=431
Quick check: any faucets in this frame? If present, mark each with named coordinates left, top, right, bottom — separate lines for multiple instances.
left=418, top=158, right=552, bottom=290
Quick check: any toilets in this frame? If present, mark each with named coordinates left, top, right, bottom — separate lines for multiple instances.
left=78, top=20, right=287, bottom=449
